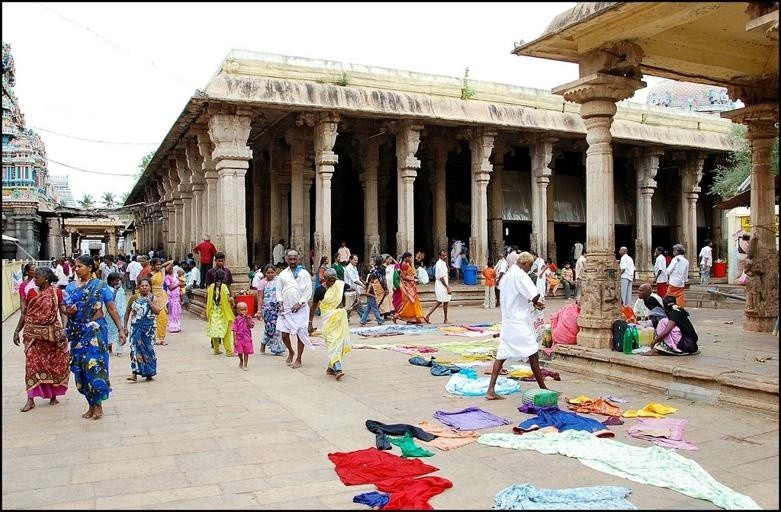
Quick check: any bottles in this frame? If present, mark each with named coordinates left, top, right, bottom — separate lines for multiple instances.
left=623, top=325, right=634, bottom=354
left=632, top=324, right=640, bottom=349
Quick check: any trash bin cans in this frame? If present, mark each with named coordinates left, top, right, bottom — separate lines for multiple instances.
left=713, top=261, right=726, bottom=277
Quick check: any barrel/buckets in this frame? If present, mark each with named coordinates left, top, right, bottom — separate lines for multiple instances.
left=712, top=262, right=726, bottom=278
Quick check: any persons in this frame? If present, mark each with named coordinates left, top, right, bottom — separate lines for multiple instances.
left=231, top=241, right=360, bottom=379
left=529, top=252, right=587, bottom=303
left=620, top=244, right=698, bottom=356
left=700, top=239, right=713, bottom=284
left=737, top=233, right=750, bottom=274
left=483, top=242, right=520, bottom=308
left=334, top=237, right=466, bottom=324
left=14, top=233, right=233, bottom=419
left=485, top=252, right=563, bottom=400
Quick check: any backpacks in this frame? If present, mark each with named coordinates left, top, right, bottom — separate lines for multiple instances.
left=612, top=319, right=636, bottom=350
